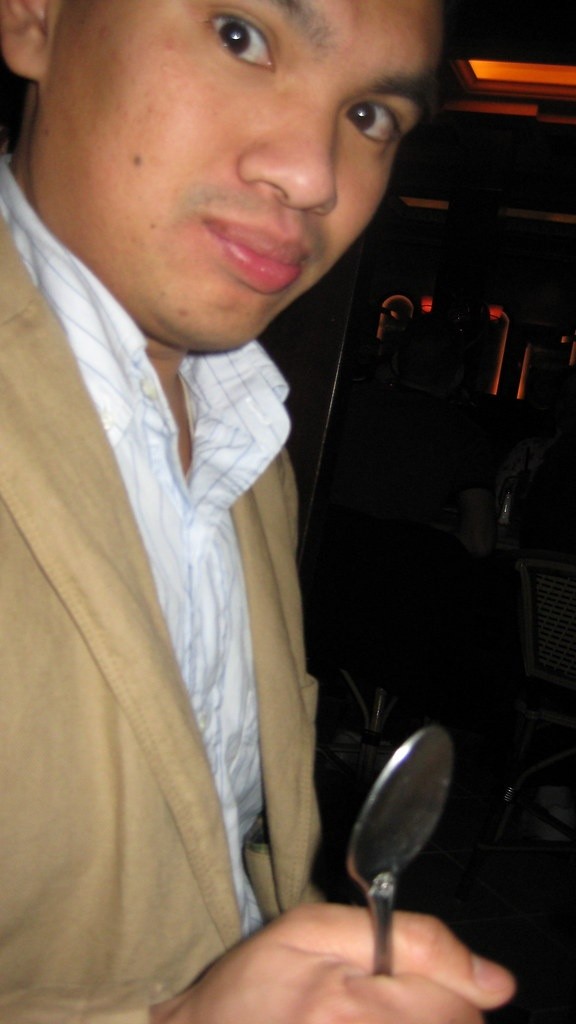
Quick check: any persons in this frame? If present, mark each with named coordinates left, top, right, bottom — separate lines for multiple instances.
left=0, top=1, right=516, bottom=1024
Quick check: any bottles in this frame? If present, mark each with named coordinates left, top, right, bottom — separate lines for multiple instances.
left=497, top=488, right=513, bottom=537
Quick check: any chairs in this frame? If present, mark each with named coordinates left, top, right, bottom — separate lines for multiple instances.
left=450, top=548, right=576, bottom=901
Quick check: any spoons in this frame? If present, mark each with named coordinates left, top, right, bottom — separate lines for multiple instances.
left=346, top=726, right=453, bottom=974
